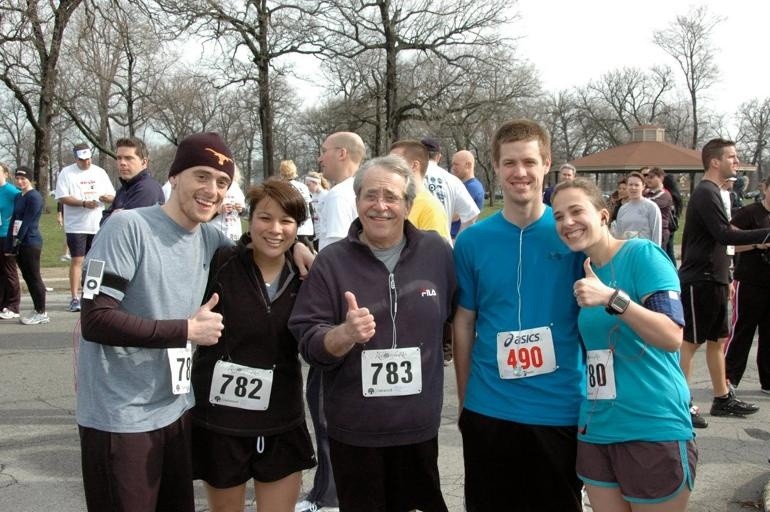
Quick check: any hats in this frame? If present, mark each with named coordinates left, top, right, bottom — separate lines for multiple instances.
left=76, top=149, right=91, bottom=160
left=15, top=166, right=32, bottom=182
left=421, top=139, right=439, bottom=151
left=645, top=167, right=664, bottom=177
left=169, top=132, right=233, bottom=190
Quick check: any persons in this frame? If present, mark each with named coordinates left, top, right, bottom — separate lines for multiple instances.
left=289, top=157, right=457, bottom=512
left=4, top=166, right=50, bottom=326
left=723, top=177, right=770, bottom=393
left=548, top=164, right=682, bottom=265
left=74, top=133, right=317, bottom=512
left=722, top=175, right=764, bottom=257
left=677, top=138, right=770, bottom=428
left=283, top=141, right=485, bottom=364
left=452, top=120, right=583, bottom=512
left=56, top=138, right=246, bottom=312
left=551, top=177, right=699, bottom=512
left=294, top=131, right=366, bottom=512
left=0, top=161, right=22, bottom=319
left=193, top=179, right=318, bottom=512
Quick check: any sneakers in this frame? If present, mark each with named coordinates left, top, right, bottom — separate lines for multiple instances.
left=0, top=309, right=50, bottom=324
left=689, top=380, right=770, bottom=428
left=295, top=499, right=340, bottom=512
left=70, top=299, right=81, bottom=311
